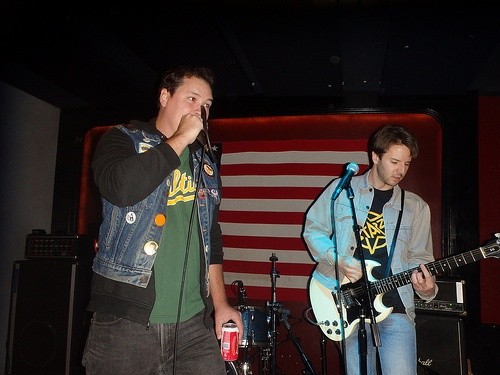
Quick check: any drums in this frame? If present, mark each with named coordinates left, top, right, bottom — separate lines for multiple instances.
left=225, top=360, right=240, bottom=375
left=234, top=305, right=273, bottom=349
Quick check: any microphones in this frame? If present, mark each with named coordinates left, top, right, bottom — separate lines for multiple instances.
left=197, top=130, right=216, bottom=164
left=239, top=282, right=247, bottom=305
left=330, top=163, right=359, bottom=200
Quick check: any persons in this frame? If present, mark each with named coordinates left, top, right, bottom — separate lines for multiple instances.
left=302, top=125, right=439, bottom=375
left=81, top=63, right=243, bottom=375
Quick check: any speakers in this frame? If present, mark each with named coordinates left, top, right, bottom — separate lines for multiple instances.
left=6, top=257, right=97, bottom=375
left=376, top=310, right=468, bottom=375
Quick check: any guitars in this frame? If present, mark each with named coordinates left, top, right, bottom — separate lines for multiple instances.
left=309, top=232, right=500, bottom=342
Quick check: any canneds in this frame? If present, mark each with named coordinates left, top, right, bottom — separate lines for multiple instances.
left=220, top=323, right=239, bottom=361
